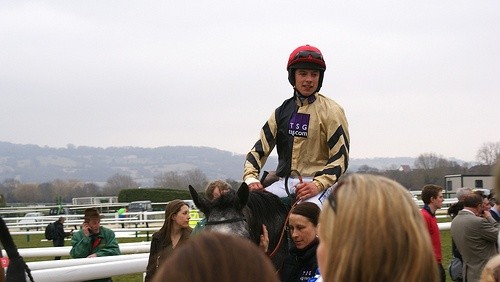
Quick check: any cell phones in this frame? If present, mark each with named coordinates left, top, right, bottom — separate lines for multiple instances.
left=84, top=222, right=93, bottom=233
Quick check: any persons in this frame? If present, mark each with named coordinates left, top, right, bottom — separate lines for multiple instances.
left=153, top=230, right=279, bottom=282
left=243, top=44, right=350, bottom=209
left=449, top=188, right=500, bottom=282
left=190, top=180, right=232, bottom=238
left=318, top=174, right=440, bottom=282
left=420, top=184, right=446, bottom=282
left=261, top=201, right=323, bottom=282
left=70, top=208, right=121, bottom=282
left=145, top=200, right=193, bottom=282
left=53, top=217, right=72, bottom=260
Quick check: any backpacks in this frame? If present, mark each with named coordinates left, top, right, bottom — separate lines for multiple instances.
left=45, top=222, right=64, bottom=240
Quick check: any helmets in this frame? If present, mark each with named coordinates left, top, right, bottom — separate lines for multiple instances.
left=286, top=45, right=326, bottom=93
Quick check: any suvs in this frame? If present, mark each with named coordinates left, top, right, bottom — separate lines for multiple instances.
left=128, top=200, right=153, bottom=224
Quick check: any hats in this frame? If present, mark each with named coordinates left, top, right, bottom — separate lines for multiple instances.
left=80, top=207, right=105, bottom=220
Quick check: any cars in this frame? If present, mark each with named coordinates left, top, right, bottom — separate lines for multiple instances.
left=19, top=213, right=45, bottom=231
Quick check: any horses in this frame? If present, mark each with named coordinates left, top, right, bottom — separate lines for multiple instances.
left=187, top=181, right=323, bottom=276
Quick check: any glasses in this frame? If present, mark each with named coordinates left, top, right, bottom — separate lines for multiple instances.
left=328, top=180, right=343, bottom=214
